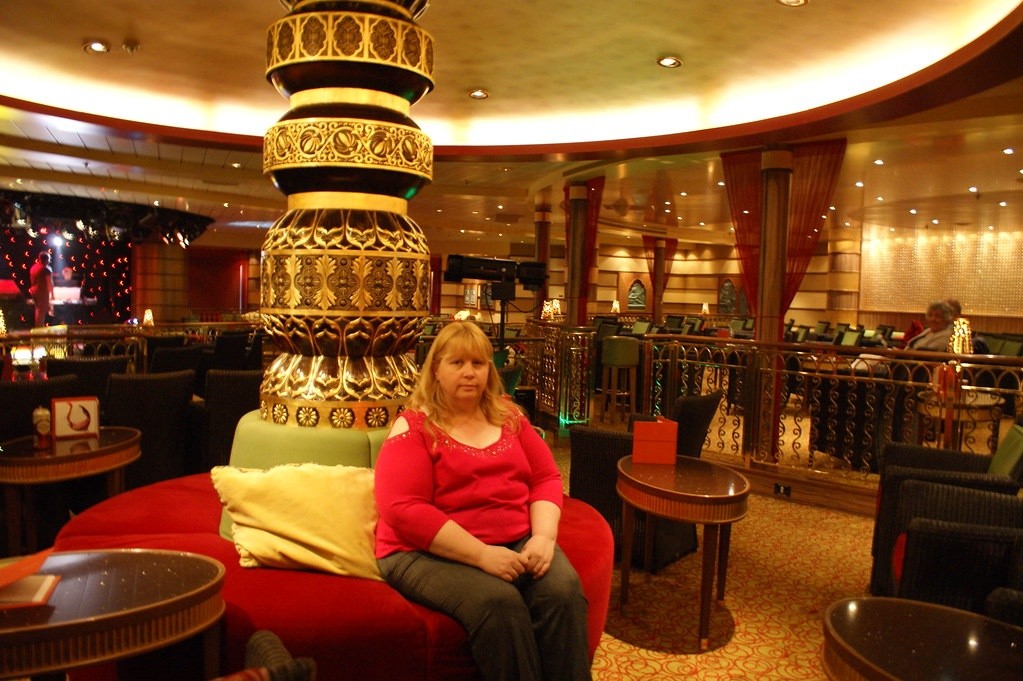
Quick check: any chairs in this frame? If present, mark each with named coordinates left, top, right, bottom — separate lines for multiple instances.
left=419, top=315, right=1023, bottom=408
left=869, top=423, right=1023, bottom=621
left=0, top=324, right=268, bottom=499
left=561, top=389, right=721, bottom=571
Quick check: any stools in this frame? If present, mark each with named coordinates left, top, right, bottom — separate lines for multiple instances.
left=596, top=335, right=640, bottom=425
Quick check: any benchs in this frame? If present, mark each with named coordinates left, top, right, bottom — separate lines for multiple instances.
left=57, top=476, right=613, bottom=680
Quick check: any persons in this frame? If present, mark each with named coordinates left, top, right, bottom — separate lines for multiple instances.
left=29, top=251, right=55, bottom=328
left=901, top=300, right=957, bottom=353
left=373, top=320, right=594, bottom=681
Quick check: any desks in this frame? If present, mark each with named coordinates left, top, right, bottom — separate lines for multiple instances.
left=0, top=548, right=225, bottom=681
left=1, top=426, right=143, bottom=563
left=614, top=455, right=750, bottom=642
left=819, top=599, right=1023, bottom=681
left=920, top=392, right=1005, bottom=450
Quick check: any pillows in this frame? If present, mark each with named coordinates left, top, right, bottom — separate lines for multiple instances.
left=210, top=460, right=384, bottom=579
